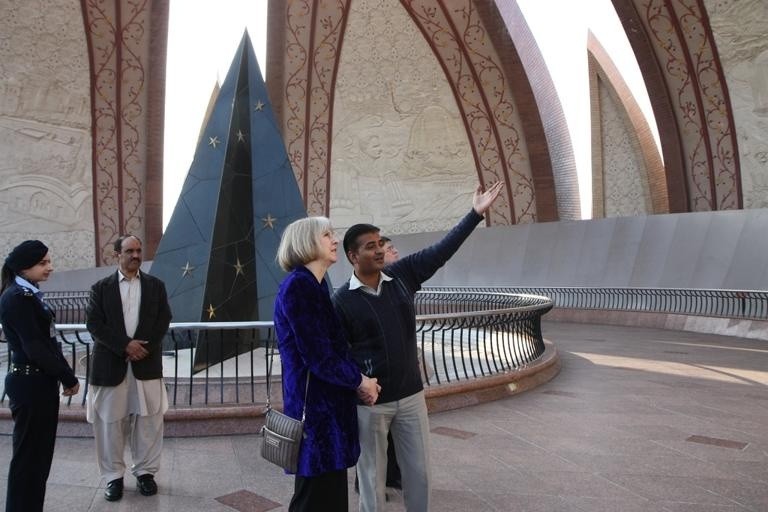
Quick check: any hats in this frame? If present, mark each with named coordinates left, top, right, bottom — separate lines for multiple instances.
left=6, top=239, right=49, bottom=271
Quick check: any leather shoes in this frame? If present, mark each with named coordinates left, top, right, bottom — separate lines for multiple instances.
left=104, top=477, right=124, bottom=502
left=136, top=474, right=157, bottom=496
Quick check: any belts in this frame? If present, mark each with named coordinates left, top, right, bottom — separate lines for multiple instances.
left=8, top=364, right=50, bottom=377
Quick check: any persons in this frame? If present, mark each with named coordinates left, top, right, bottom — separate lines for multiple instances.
left=352, top=234, right=403, bottom=504
left=0, top=237, right=81, bottom=512
left=328, top=178, right=509, bottom=512
left=80, top=233, right=173, bottom=504
left=268, top=214, right=384, bottom=512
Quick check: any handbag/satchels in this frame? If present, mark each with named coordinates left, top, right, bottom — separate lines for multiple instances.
left=261, top=409, right=303, bottom=474
